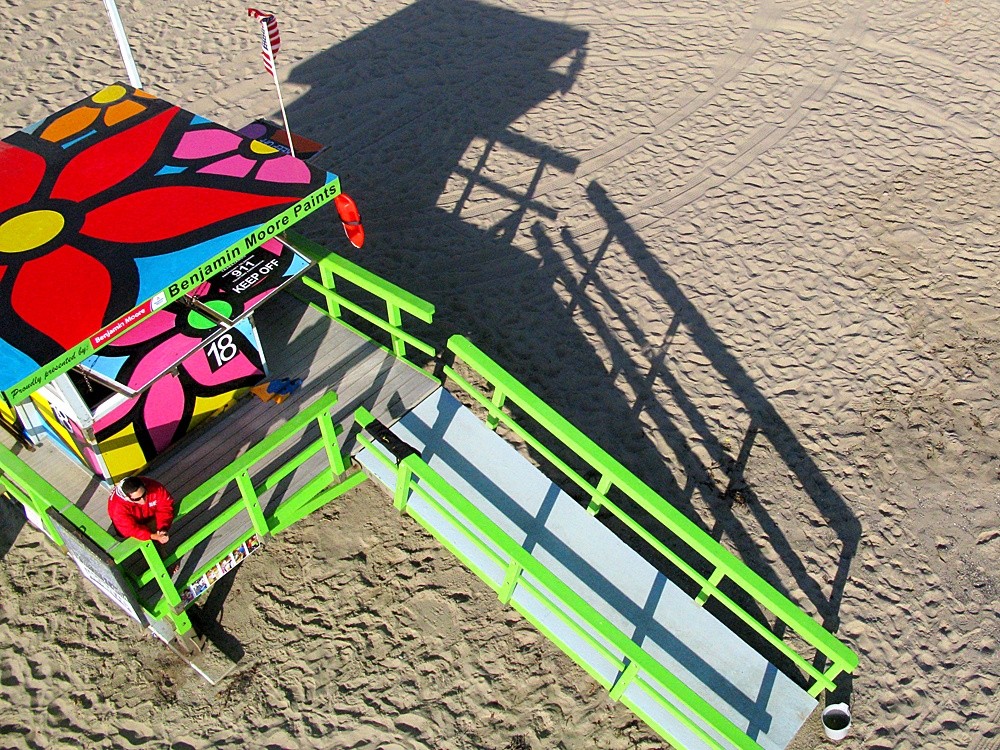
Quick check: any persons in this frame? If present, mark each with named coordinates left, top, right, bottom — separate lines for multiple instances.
left=107, top=476, right=175, bottom=544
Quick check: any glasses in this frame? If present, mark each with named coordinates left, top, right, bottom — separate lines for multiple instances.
left=128, top=491, right=146, bottom=502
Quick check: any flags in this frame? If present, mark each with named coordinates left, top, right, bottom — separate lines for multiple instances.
left=247, top=8, right=280, bottom=76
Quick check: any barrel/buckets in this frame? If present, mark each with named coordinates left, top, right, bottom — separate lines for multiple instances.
left=820, top=702, right=852, bottom=740
